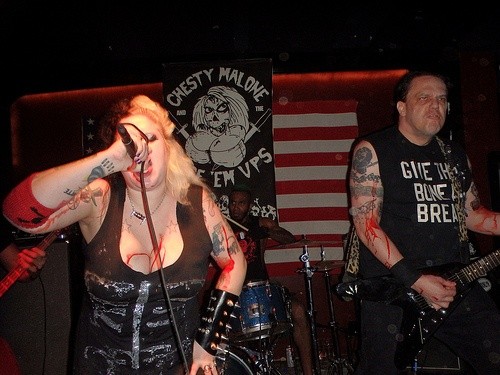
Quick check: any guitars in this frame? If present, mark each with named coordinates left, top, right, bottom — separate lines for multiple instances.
left=335, top=248, right=500, bottom=375
left=0, top=228, right=64, bottom=298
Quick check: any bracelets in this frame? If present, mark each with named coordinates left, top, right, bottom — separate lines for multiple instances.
left=390, top=256, right=422, bottom=287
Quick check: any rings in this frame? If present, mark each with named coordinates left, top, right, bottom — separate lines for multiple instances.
left=213, top=360, right=217, bottom=367
left=203, top=365, right=211, bottom=373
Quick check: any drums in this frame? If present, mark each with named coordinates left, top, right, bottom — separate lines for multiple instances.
left=223, top=347, right=258, bottom=375
left=236, top=280, right=278, bottom=339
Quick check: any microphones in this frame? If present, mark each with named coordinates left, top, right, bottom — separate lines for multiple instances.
left=117, top=123, right=143, bottom=164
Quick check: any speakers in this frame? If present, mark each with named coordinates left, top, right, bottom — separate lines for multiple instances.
left=0, top=240, right=89, bottom=375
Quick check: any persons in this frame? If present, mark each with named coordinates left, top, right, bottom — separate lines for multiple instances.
left=347, top=67, right=500, bottom=375
left=225, top=190, right=312, bottom=375
left=3, top=96, right=247, bottom=375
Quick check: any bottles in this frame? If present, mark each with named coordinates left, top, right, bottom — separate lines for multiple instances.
left=286, top=345, right=294, bottom=367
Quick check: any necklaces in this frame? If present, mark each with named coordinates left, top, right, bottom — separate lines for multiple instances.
left=127, top=188, right=166, bottom=225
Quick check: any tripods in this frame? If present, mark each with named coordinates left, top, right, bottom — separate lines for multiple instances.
left=324, top=271, right=355, bottom=375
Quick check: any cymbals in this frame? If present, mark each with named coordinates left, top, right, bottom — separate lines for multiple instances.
left=296, top=261, right=345, bottom=273
left=265, top=239, right=347, bottom=250
